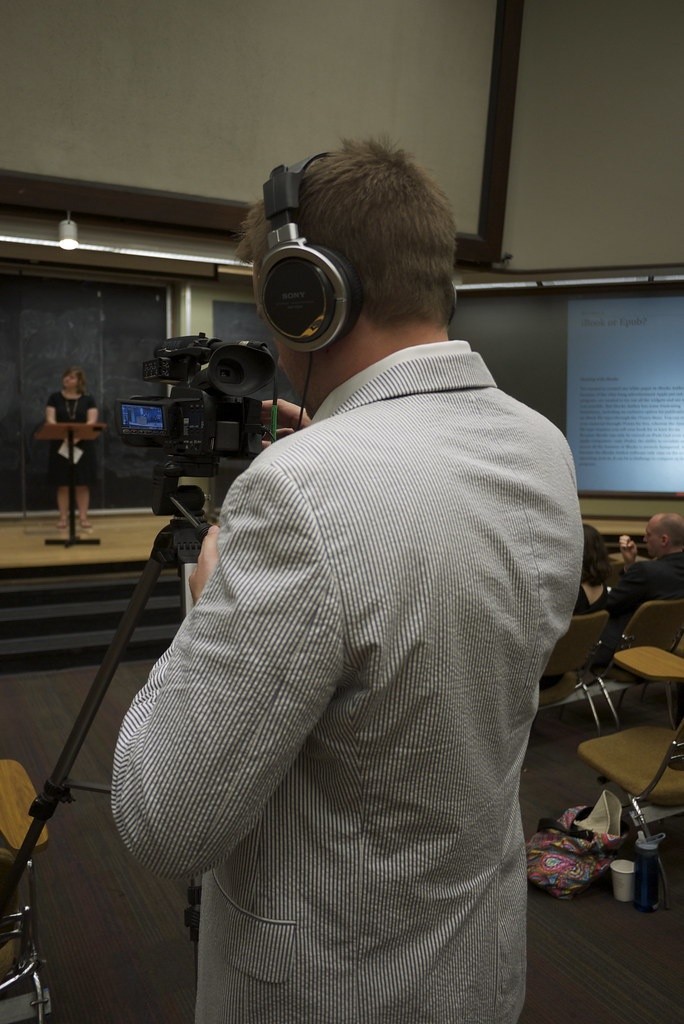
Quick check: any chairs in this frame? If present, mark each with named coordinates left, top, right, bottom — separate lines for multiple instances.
left=0, top=758, right=51, bottom=1024
left=538, top=610, right=610, bottom=738
left=614, top=646, right=684, bottom=730
left=535, top=719, right=684, bottom=912
left=594, top=599, right=684, bottom=730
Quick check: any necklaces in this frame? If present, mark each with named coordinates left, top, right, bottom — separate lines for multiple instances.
left=64, top=394, right=79, bottom=420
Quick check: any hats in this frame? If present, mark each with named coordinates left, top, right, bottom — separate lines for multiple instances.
left=577, top=790, right=622, bottom=836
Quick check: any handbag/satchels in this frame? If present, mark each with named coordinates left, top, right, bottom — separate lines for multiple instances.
left=526, top=806, right=631, bottom=900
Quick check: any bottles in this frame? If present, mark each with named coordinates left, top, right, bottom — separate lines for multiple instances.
left=635, top=831, right=665, bottom=913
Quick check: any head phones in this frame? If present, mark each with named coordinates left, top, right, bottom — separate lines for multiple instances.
left=258, top=151, right=363, bottom=352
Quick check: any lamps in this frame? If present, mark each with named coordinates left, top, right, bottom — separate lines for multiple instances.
left=59, top=211, right=79, bottom=250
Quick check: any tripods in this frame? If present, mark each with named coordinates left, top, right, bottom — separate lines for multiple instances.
left=0, top=451, right=232, bottom=974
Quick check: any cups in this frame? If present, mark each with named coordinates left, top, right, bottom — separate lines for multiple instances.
left=610, top=860, right=635, bottom=901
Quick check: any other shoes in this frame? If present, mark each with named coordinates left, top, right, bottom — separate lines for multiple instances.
left=56, top=518, right=67, bottom=530
left=80, top=519, right=93, bottom=529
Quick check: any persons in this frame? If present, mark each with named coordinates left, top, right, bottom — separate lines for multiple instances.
left=111, top=138, right=584, bottom=1024
left=46, top=366, right=98, bottom=528
left=539, top=523, right=614, bottom=694
left=585, top=512, right=684, bottom=675
